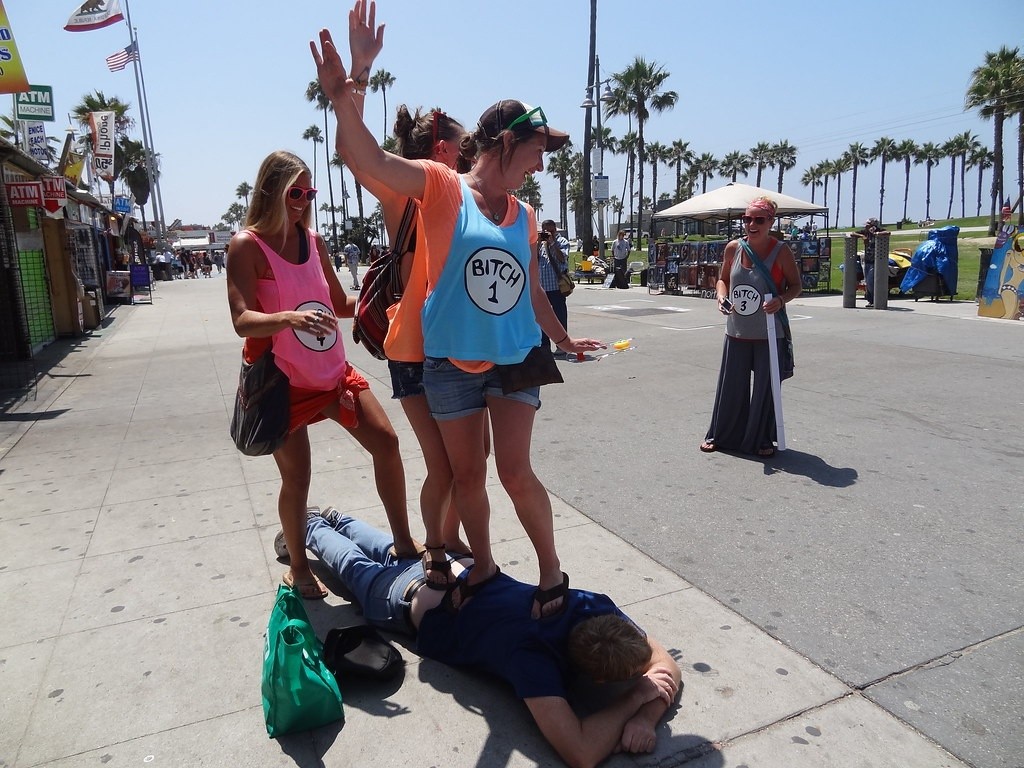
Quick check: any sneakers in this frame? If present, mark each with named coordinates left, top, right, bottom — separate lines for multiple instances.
left=274, top=506, right=321, bottom=559
left=321, top=505, right=342, bottom=525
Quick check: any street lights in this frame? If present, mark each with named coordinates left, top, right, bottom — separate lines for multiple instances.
left=64, top=124, right=80, bottom=152
left=580, top=78, right=618, bottom=261
left=342, top=189, right=350, bottom=236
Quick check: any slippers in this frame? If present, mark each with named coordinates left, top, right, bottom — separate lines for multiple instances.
left=451, top=563, right=501, bottom=610
left=700, top=437, right=717, bottom=452
left=530, top=571, right=569, bottom=623
left=422, top=550, right=458, bottom=590
left=282, top=569, right=328, bottom=600
left=444, top=546, right=474, bottom=558
left=388, top=539, right=427, bottom=559
left=758, top=446, right=775, bottom=458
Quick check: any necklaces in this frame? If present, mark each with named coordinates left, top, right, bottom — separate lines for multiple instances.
left=467, top=171, right=507, bottom=220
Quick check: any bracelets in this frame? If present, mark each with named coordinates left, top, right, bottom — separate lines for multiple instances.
left=776, top=295, right=786, bottom=308
left=876, top=232, right=878, bottom=237
left=555, top=335, right=568, bottom=344
left=352, top=88, right=367, bottom=96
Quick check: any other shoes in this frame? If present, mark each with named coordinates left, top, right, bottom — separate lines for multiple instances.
left=554, top=348, right=565, bottom=355
left=350, top=284, right=361, bottom=290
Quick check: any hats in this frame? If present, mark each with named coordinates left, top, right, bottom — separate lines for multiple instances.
left=475, top=99, right=569, bottom=152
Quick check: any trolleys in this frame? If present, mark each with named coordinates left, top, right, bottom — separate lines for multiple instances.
left=573, top=254, right=607, bottom=284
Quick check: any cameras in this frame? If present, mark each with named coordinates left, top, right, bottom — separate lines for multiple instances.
left=538, top=233, right=548, bottom=241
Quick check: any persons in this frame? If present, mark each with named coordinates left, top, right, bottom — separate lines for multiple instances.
left=155, top=247, right=230, bottom=280
left=575, top=230, right=633, bottom=288
left=226, top=151, right=425, bottom=600
left=787, top=222, right=817, bottom=240
left=334, top=0, right=491, bottom=591
left=537, top=220, right=569, bottom=355
left=699, top=196, right=803, bottom=459
left=851, top=217, right=891, bottom=307
left=274, top=506, right=681, bottom=768
left=311, top=29, right=607, bottom=623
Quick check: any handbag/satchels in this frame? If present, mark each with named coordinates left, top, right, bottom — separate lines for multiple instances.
left=324, top=624, right=403, bottom=691
left=558, top=269, right=575, bottom=297
left=351, top=250, right=404, bottom=361
left=496, top=341, right=565, bottom=397
left=230, top=352, right=291, bottom=456
left=261, top=585, right=345, bottom=737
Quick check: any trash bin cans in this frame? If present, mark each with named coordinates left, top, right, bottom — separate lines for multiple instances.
left=897, top=222, right=902, bottom=229
left=976, top=248, right=994, bottom=300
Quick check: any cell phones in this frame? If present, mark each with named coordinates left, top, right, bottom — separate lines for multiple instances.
left=721, top=299, right=732, bottom=313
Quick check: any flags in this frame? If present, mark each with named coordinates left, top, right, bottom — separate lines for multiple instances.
left=63, top=0, right=124, bottom=32
left=106, top=40, right=140, bottom=72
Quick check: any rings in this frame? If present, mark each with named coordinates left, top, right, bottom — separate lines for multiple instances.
left=315, top=317, right=319, bottom=322
left=316, top=310, right=322, bottom=317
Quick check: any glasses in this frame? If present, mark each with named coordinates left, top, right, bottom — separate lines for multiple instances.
left=286, top=186, right=318, bottom=202
left=741, top=215, right=769, bottom=225
left=432, top=111, right=446, bottom=147
left=508, top=106, right=547, bottom=135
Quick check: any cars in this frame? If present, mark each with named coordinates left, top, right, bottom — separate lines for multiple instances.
left=621, top=228, right=650, bottom=239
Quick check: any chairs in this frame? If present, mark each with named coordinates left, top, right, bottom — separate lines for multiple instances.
left=629, top=261, right=644, bottom=284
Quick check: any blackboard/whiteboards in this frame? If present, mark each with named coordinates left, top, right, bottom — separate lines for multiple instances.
left=130, top=264, right=150, bottom=287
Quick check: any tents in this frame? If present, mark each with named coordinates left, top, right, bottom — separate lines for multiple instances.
left=648, top=182, right=830, bottom=294
